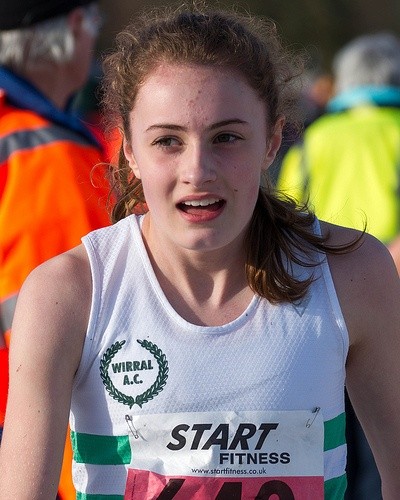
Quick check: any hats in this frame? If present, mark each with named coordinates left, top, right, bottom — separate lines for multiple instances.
left=0, top=0, right=92, bottom=30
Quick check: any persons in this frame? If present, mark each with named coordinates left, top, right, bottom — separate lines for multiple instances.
left=0, top=0, right=400, bottom=500
left=0, top=1, right=400, bottom=500
